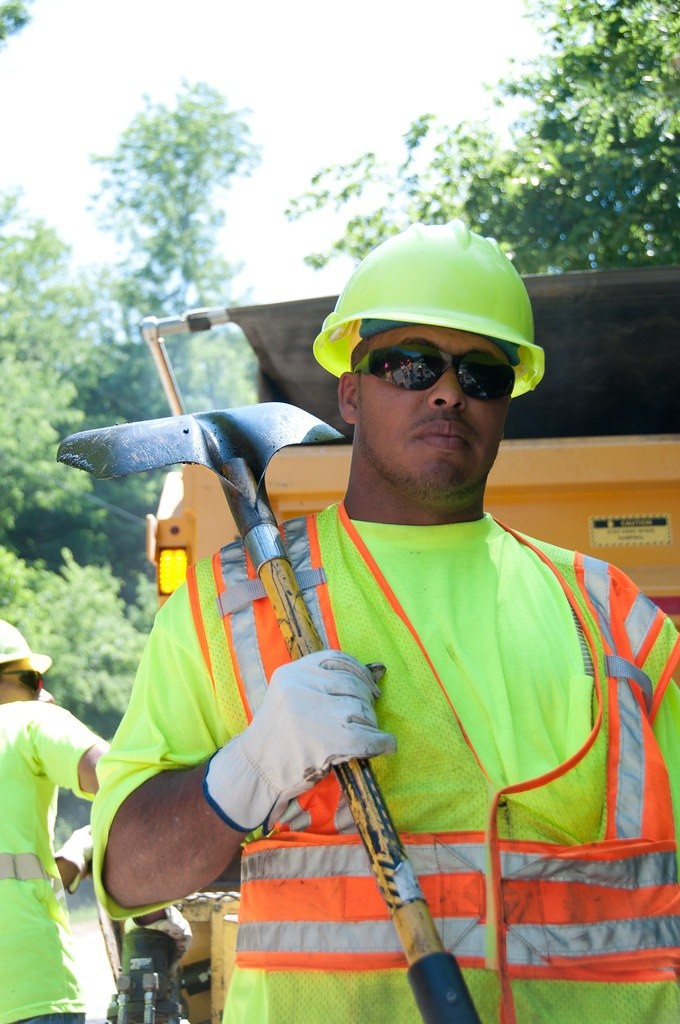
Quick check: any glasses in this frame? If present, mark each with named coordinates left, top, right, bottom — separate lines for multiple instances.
left=347, top=344, right=518, bottom=403
left=0, top=670, right=44, bottom=692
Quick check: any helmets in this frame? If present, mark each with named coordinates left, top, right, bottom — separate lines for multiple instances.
left=0, top=617, right=53, bottom=675
left=312, top=219, right=544, bottom=399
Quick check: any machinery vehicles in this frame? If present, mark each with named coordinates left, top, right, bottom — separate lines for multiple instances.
left=136, top=259, right=679, bottom=672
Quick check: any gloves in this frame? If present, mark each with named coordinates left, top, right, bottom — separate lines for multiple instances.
left=198, top=648, right=399, bottom=832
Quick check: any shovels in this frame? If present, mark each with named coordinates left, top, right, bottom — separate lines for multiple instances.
left=51, top=395, right=484, bottom=1024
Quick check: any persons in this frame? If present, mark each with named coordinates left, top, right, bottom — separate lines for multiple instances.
left=90, top=220, right=680, bottom=1023
left=0, top=618, right=110, bottom=1024
